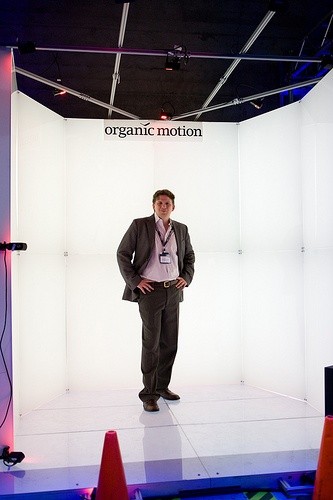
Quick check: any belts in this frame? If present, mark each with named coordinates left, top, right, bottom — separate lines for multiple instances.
left=148, top=279, right=179, bottom=288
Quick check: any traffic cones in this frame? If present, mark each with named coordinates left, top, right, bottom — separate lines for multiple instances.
left=313, top=415, right=333, bottom=500
left=94, top=431, right=130, bottom=500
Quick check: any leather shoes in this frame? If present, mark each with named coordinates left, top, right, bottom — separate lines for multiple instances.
left=158, top=388, right=180, bottom=401
left=143, top=400, right=159, bottom=412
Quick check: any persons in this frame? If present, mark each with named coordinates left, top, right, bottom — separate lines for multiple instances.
left=117, top=189, right=195, bottom=411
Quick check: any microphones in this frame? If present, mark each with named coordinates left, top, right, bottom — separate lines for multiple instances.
left=0, top=242, right=27, bottom=251
